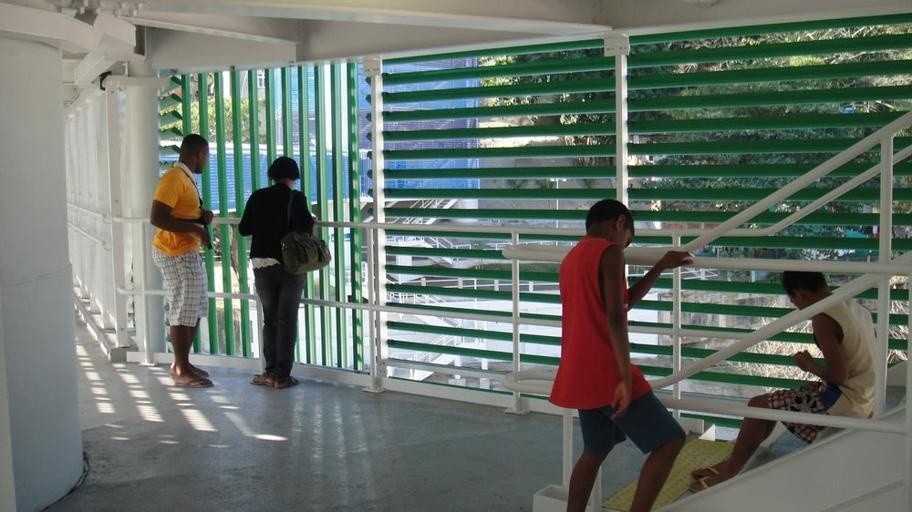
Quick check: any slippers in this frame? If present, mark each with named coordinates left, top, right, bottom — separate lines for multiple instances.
left=250, top=375, right=273, bottom=385
left=275, top=376, right=297, bottom=389
left=690, top=466, right=719, bottom=492
left=170, top=368, right=213, bottom=387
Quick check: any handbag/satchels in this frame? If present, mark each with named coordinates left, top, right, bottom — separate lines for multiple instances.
left=281, top=232, right=331, bottom=275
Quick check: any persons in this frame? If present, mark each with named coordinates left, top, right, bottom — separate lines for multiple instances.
left=547, top=198, right=696, bottom=511
left=148, top=133, right=217, bottom=391
left=688, top=269, right=878, bottom=493
left=239, top=154, right=314, bottom=391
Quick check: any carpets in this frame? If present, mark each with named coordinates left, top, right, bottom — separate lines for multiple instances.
left=601, top=435, right=733, bottom=511
